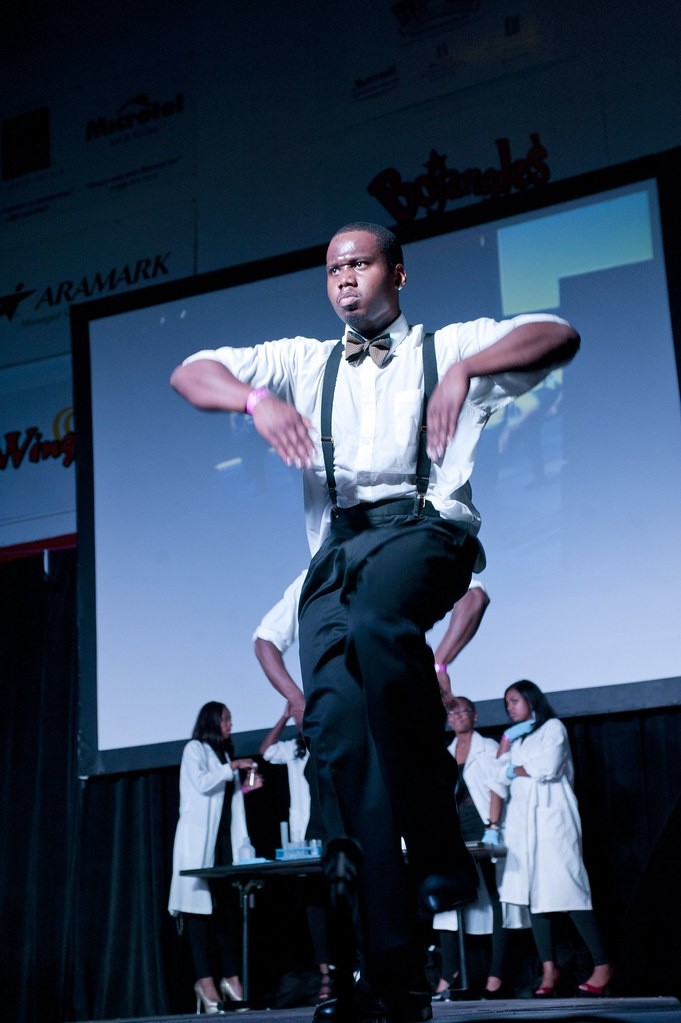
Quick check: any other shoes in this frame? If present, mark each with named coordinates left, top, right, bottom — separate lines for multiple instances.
left=417, top=838, right=479, bottom=917
left=312, top=968, right=432, bottom=1023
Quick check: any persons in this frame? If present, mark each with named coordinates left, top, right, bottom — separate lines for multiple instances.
left=169, top=220, right=582, bottom=1022
left=252, top=569, right=490, bottom=1005
left=479, top=679, right=615, bottom=994
left=169, top=701, right=264, bottom=1015
left=259, top=702, right=311, bottom=842
left=431, top=697, right=532, bottom=1000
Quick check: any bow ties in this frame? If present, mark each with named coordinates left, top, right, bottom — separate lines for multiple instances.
left=345, top=331, right=392, bottom=368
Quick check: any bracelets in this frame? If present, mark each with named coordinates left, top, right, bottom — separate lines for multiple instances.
left=247, top=387, right=272, bottom=413
left=436, top=663, right=447, bottom=673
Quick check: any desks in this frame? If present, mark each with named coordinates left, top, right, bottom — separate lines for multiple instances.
left=177, top=847, right=501, bottom=1011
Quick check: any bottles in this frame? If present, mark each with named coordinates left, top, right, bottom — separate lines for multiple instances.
left=246, top=762, right=258, bottom=789
left=236, top=836, right=255, bottom=864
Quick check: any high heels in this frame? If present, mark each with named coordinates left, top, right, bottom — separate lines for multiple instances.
left=479, top=975, right=511, bottom=1000
left=432, top=972, right=461, bottom=1002
left=579, top=970, right=613, bottom=998
left=220, top=976, right=249, bottom=1012
left=535, top=968, right=561, bottom=997
left=195, top=981, right=227, bottom=1016
